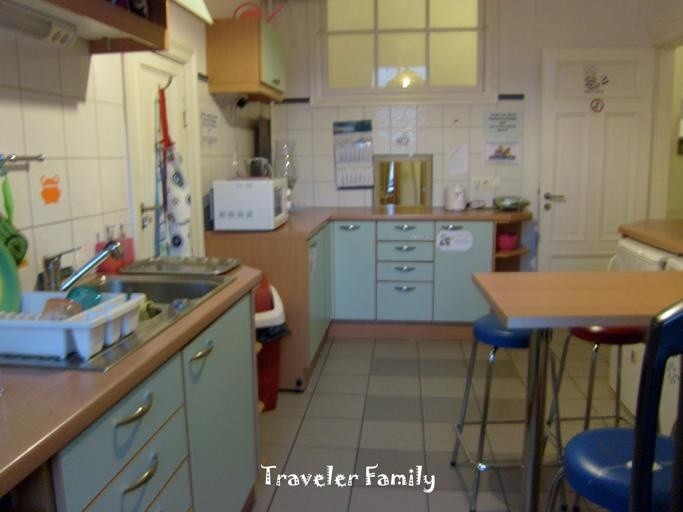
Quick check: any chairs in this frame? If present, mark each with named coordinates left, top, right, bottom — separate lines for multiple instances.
left=545, top=297, right=683, bottom=511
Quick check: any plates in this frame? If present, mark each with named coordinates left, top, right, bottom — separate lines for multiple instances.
left=493, top=196, right=530, bottom=210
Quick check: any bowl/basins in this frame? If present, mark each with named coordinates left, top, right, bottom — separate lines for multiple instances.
left=495, top=234, right=517, bottom=251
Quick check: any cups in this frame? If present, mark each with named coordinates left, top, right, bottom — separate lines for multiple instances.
left=387, top=204, right=395, bottom=215
left=39, top=284, right=100, bottom=321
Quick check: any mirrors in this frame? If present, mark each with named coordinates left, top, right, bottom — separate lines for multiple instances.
left=370, top=154, right=434, bottom=209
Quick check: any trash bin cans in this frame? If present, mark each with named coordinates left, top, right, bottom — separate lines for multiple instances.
left=254, top=273, right=291, bottom=412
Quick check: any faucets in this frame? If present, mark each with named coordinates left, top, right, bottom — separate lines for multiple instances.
left=55, top=238, right=124, bottom=299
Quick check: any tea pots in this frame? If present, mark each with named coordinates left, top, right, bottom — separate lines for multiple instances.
left=444, top=185, right=467, bottom=212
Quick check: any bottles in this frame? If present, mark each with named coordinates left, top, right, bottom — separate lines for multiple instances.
left=274, top=140, right=296, bottom=186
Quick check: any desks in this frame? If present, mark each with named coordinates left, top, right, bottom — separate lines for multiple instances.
left=468, top=266, right=682, bottom=511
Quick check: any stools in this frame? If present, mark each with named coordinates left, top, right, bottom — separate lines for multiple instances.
left=450, top=311, right=569, bottom=512
left=540, top=322, right=645, bottom=508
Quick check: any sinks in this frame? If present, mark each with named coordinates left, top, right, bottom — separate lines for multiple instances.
left=77, top=276, right=223, bottom=306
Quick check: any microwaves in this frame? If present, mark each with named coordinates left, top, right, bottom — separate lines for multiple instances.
left=211, top=178, right=288, bottom=231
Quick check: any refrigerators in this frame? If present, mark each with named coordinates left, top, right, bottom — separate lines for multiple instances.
left=607, top=234, right=675, bottom=422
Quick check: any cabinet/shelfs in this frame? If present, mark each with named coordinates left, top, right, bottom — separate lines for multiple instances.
left=0, top=352, right=195, bottom=512
left=205, top=15, right=285, bottom=100
left=493, top=214, right=530, bottom=272
left=1, top=1, right=170, bottom=55
left=204, top=215, right=332, bottom=393
left=178, top=289, right=266, bottom=512
left=331, top=218, right=375, bottom=325
left=375, top=218, right=496, bottom=324
